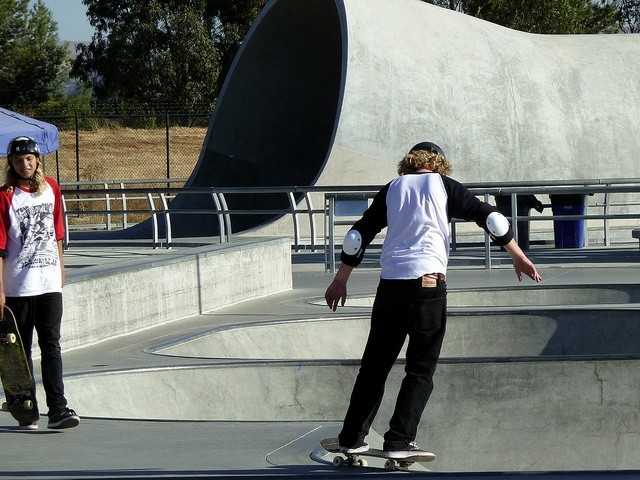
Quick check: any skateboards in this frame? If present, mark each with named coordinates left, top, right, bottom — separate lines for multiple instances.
left=320, top=438, right=437, bottom=471
left=0, top=305, right=40, bottom=424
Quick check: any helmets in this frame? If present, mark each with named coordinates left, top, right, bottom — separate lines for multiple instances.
left=7, top=135, right=42, bottom=165
left=409, top=142, right=446, bottom=160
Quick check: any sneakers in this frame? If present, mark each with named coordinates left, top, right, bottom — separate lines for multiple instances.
left=47, top=407, right=81, bottom=429
left=383, top=441, right=436, bottom=459
left=340, top=434, right=369, bottom=455
left=18, top=421, right=39, bottom=430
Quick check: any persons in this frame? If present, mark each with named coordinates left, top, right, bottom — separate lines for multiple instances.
left=0, top=136, right=80, bottom=431
left=324, top=142, right=543, bottom=462
